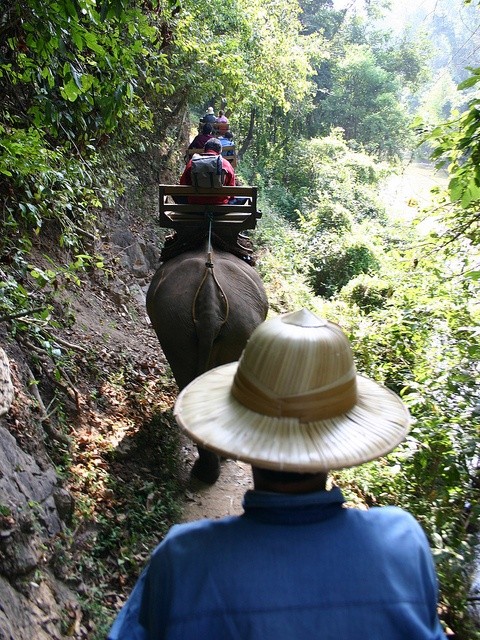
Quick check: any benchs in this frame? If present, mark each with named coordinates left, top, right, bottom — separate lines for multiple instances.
left=186, top=145, right=237, bottom=169
left=198, top=122, right=230, bottom=135
left=158, top=183, right=263, bottom=256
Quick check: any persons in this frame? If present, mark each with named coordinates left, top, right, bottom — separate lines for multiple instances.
left=104, top=310, right=446, bottom=640
left=180, top=138, right=251, bottom=236
left=215, top=110, right=229, bottom=124
left=187, top=123, right=215, bottom=160
left=202, top=107, right=216, bottom=123
left=217, top=131, right=235, bottom=163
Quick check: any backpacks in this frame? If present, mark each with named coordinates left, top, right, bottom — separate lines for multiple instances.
left=189, top=155, right=226, bottom=190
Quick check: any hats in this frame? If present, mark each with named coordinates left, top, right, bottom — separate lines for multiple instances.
left=206, top=107, right=215, bottom=115
left=174, top=311, right=411, bottom=473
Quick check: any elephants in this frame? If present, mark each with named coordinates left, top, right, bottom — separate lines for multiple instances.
left=146, top=246, right=269, bottom=486
